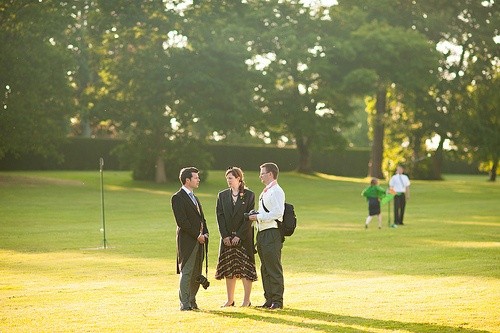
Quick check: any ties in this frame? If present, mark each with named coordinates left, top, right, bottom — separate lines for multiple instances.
left=399, top=175, right=405, bottom=188
left=189, top=193, right=197, bottom=208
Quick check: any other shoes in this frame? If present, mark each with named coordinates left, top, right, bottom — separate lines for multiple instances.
left=269, top=303, right=283, bottom=309
left=257, top=301, right=270, bottom=308
left=221, top=301, right=235, bottom=307
left=242, top=302, right=252, bottom=307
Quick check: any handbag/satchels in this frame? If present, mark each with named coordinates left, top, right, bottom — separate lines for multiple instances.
left=277, top=202, right=297, bottom=237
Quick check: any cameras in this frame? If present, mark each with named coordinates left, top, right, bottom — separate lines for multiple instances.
left=244, top=210, right=259, bottom=222
left=196, top=275, right=210, bottom=289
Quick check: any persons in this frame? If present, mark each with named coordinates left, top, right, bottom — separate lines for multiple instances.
left=249, top=162, right=285, bottom=309
left=389, top=165, right=411, bottom=225
left=215, top=167, right=258, bottom=308
left=171, top=167, right=210, bottom=312
left=363, top=178, right=386, bottom=229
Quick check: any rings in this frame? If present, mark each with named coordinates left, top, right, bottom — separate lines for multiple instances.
left=200, top=242, right=201, bottom=243
left=225, top=242, right=227, bottom=244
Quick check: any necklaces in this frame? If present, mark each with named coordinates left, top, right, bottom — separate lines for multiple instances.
left=232, top=193, right=238, bottom=197
